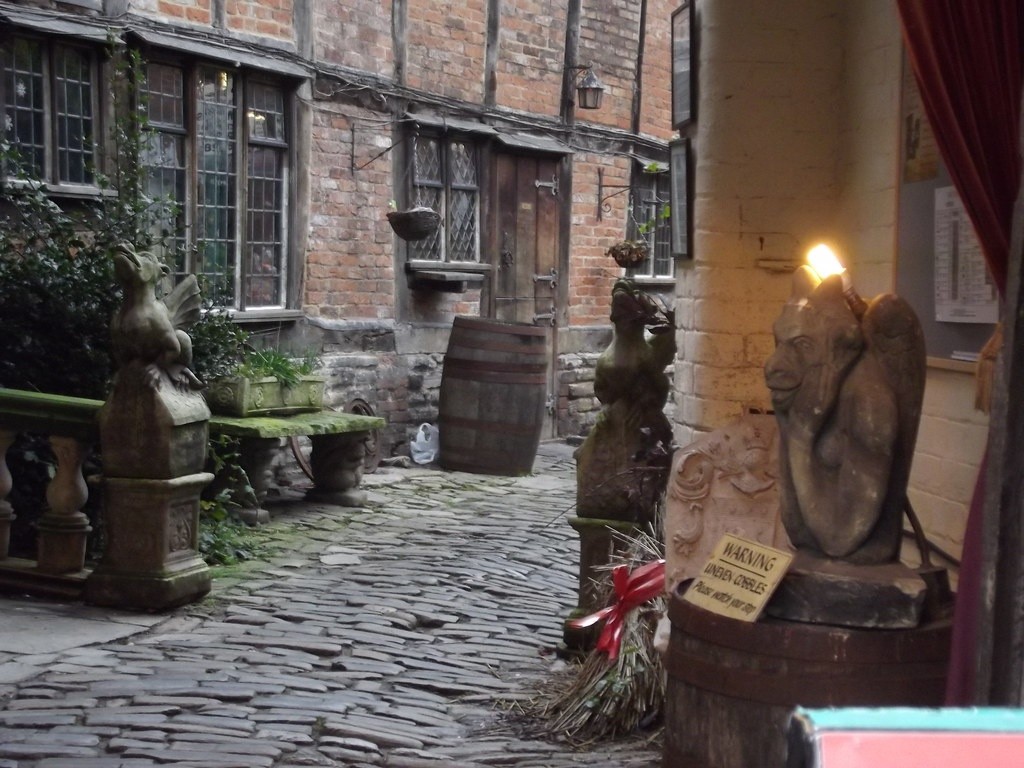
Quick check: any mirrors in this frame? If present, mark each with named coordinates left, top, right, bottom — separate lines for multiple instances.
left=671, top=0, right=695, bottom=130
left=669, top=137, right=692, bottom=259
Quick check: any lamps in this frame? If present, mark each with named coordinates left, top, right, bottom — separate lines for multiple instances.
left=563, top=61, right=605, bottom=109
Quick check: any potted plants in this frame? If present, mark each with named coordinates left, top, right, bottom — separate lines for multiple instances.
left=201, top=346, right=326, bottom=417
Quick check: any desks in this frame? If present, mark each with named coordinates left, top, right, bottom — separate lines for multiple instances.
left=209, top=410, right=384, bottom=524
